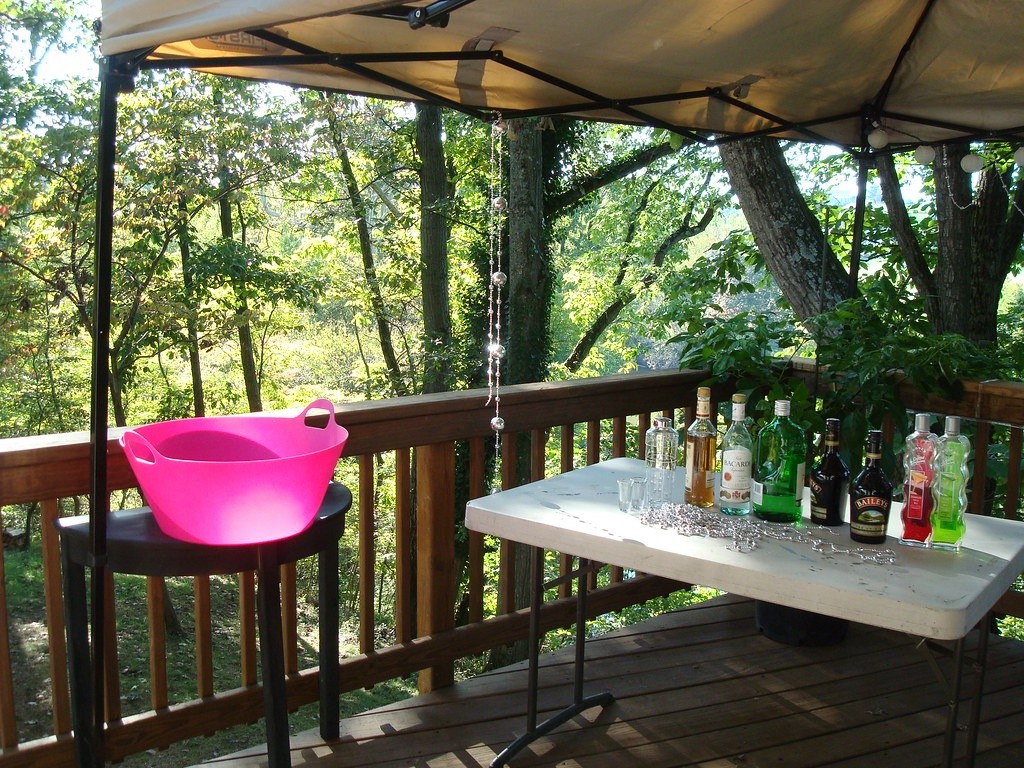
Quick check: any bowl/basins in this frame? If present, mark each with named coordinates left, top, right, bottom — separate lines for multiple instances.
left=120, top=400, right=348, bottom=547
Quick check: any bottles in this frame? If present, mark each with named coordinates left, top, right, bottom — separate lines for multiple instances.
left=685, top=387, right=716, bottom=508
left=850, top=429, right=892, bottom=544
left=751, top=400, right=805, bottom=523
left=809, top=418, right=850, bottom=526
left=927, top=415, right=970, bottom=551
left=719, top=394, right=752, bottom=516
left=645, top=418, right=679, bottom=509
left=898, top=413, right=943, bottom=549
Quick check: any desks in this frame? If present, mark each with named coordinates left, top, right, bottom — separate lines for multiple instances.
left=465, top=456, right=1024, bottom=768
left=52, top=479, right=353, bottom=768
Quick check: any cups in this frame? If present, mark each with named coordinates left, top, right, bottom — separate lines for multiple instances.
left=630, top=476, right=646, bottom=511
left=618, top=478, right=633, bottom=512
